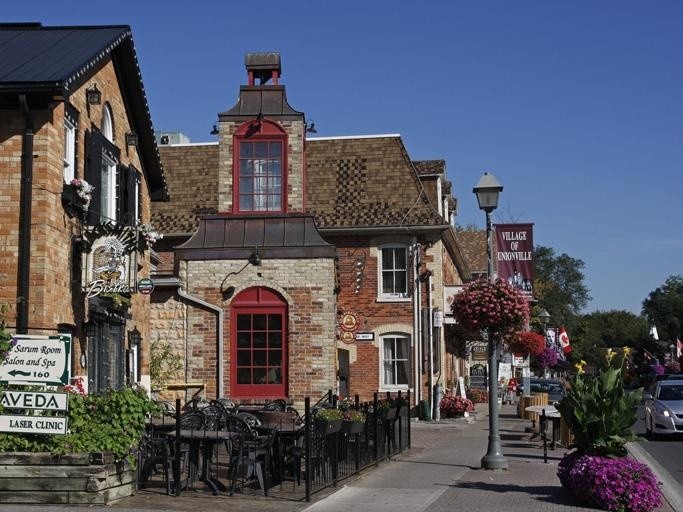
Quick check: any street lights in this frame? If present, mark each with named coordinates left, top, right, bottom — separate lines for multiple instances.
left=472, top=168, right=509, bottom=469
left=534, top=307, right=551, bottom=379
left=669, top=342, right=676, bottom=364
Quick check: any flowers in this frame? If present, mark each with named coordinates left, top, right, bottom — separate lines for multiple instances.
left=315, top=407, right=366, bottom=422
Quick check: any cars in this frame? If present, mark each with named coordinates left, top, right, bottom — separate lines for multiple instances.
left=645, top=374, right=683, bottom=395
left=640, top=377, right=683, bottom=439
left=516, top=379, right=566, bottom=419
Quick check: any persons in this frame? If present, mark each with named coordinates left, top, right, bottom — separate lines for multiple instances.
left=545, top=329, right=557, bottom=350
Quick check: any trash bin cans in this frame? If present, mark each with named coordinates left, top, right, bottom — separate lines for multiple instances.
left=520, top=393, right=548, bottom=420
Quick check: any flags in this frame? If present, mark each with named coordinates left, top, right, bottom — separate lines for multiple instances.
left=493, top=222, right=535, bottom=303
left=558, top=327, right=573, bottom=354
left=648, top=320, right=658, bottom=340
left=675, top=336, right=683, bottom=358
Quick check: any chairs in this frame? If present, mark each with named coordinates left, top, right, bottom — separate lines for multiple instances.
left=136, top=394, right=305, bottom=497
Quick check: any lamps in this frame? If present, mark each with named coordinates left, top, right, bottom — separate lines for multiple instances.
left=125, top=125, right=138, bottom=146
left=129, top=325, right=141, bottom=344
left=219, top=241, right=262, bottom=301
left=85, top=83, right=101, bottom=105
